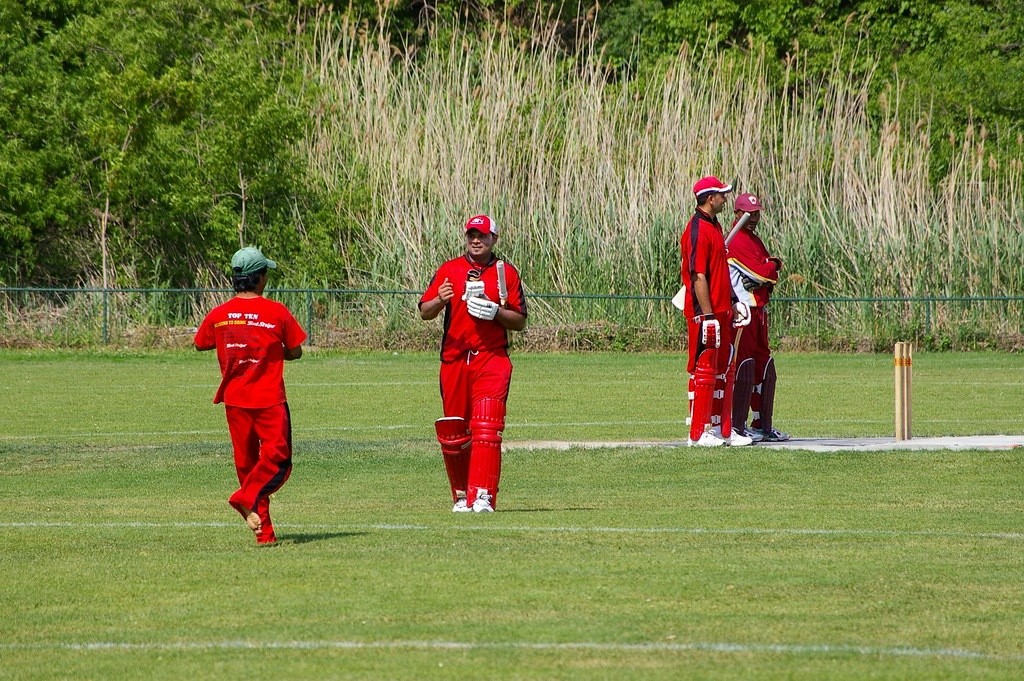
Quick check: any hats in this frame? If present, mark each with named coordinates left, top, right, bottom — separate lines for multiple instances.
left=464, top=214, right=499, bottom=237
left=735, top=194, right=765, bottom=212
left=693, top=176, right=732, bottom=198
left=230, top=248, right=276, bottom=276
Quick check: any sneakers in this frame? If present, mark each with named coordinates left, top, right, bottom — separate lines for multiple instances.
left=732, top=425, right=764, bottom=444
left=686, top=432, right=726, bottom=446
left=752, top=426, right=790, bottom=442
left=711, top=427, right=752, bottom=446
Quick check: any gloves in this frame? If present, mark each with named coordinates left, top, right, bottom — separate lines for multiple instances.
left=731, top=301, right=752, bottom=328
left=692, top=312, right=720, bottom=349
left=740, top=273, right=762, bottom=292
left=468, top=291, right=500, bottom=321
left=765, top=257, right=783, bottom=271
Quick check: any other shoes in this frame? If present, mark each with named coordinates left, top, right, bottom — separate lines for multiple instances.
left=473, top=498, right=492, bottom=513
left=452, top=500, right=473, bottom=512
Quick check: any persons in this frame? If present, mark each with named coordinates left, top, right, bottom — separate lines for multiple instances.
left=680, top=177, right=791, bottom=446
left=194, top=247, right=307, bottom=544
left=418, top=216, right=528, bottom=513
